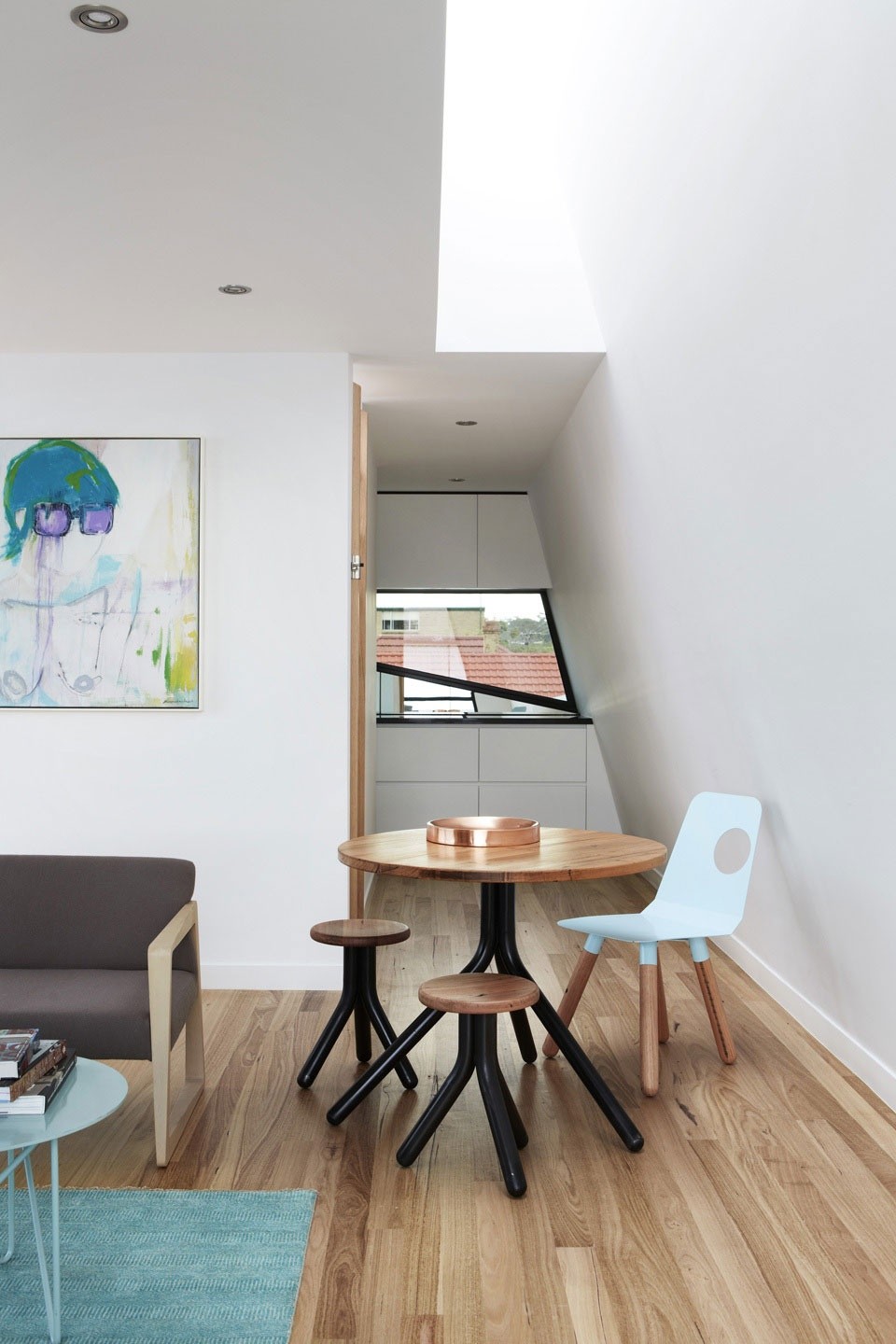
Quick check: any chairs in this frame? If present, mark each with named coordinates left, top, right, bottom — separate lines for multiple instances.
left=541, top=790, right=763, bottom=1097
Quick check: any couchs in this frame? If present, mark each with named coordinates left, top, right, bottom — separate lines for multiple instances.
left=0, top=854, right=206, bottom=1168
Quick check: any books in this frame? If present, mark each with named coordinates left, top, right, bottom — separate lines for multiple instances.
left=0, top=1047, right=79, bottom=1115
left=0, top=1027, right=41, bottom=1079
left=0, top=1040, right=67, bottom=1103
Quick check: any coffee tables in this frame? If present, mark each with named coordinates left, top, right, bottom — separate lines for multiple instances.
left=0, top=1054, right=131, bottom=1344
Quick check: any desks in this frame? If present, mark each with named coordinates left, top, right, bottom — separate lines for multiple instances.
left=339, top=826, right=668, bottom=1153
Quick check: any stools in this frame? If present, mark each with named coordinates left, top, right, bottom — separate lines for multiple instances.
left=297, top=917, right=420, bottom=1091
left=396, top=973, right=542, bottom=1199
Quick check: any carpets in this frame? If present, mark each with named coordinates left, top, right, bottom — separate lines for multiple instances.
left=1, top=1187, right=318, bottom=1344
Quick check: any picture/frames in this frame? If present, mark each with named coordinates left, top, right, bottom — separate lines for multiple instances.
left=0, top=434, right=204, bottom=714
